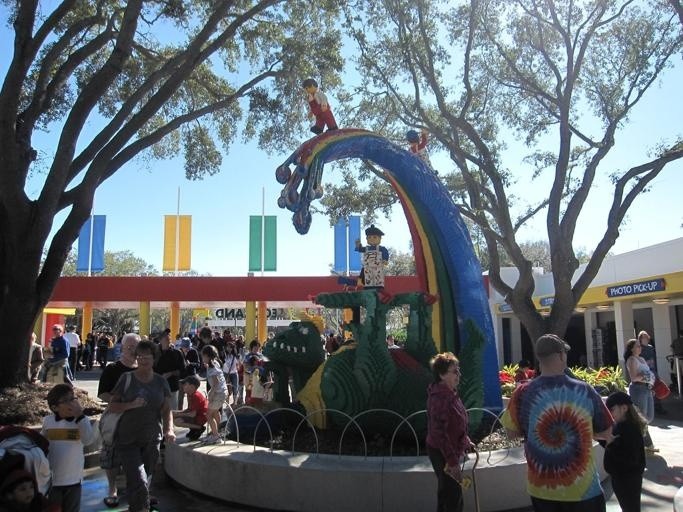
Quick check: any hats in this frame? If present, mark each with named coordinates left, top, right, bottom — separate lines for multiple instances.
left=179, top=375, right=200, bottom=389
left=533, top=333, right=571, bottom=355
left=606, top=390, right=633, bottom=409
left=0, top=468, right=34, bottom=493
left=178, top=336, right=194, bottom=348
left=158, top=327, right=171, bottom=340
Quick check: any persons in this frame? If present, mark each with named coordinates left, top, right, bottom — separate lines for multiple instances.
left=622, top=339, right=660, bottom=452
left=319, top=333, right=352, bottom=356
left=300, top=78, right=337, bottom=134
left=385, top=335, right=399, bottom=348
left=354, top=224, right=389, bottom=291
left=513, top=360, right=531, bottom=385
left=506, top=333, right=614, bottom=512
left=424, top=351, right=474, bottom=511
left=636, top=330, right=666, bottom=416
left=405, top=128, right=438, bottom=176
left=596, top=393, right=651, bottom=512
left=106, top=340, right=176, bottom=512
left=40, top=382, right=97, bottom=512
left=0, top=467, right=57, bottom=512
left=96, top=333, right=159, bottom=508
left=27, top=322, right=265, bottom=447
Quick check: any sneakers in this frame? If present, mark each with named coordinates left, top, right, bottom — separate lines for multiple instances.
left=149, top=494, right=158, bottom=506
left=644, top=444, right=660, bottom=452
left=185, top=426, right=222, bottom=445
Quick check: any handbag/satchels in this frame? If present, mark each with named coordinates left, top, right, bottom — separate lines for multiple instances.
left=224, top=373, right=233, bottom=395
left=99, top=372, right=131, bottom=444
left=653, top=376, right=671, bottom=400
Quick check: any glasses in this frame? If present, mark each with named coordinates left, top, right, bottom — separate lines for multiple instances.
left=55, top=397, right=79, bottom=405
left=445, top=366, right=461, bottom=377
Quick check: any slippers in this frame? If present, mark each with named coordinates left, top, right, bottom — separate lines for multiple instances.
left=104, top=493, right=120, bottom=507
